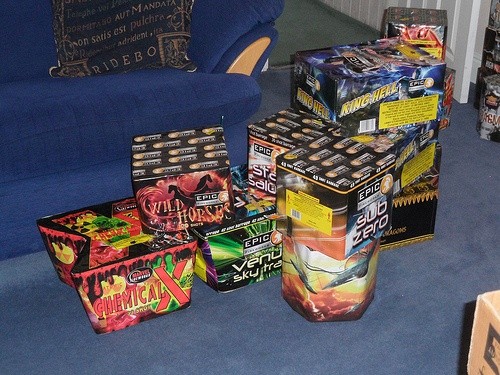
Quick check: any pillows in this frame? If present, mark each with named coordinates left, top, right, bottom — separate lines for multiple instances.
left=48, top=0, right=198, bottom=80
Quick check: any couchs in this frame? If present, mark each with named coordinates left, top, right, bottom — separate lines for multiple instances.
left=1, top=0, right=284, bottom=268
